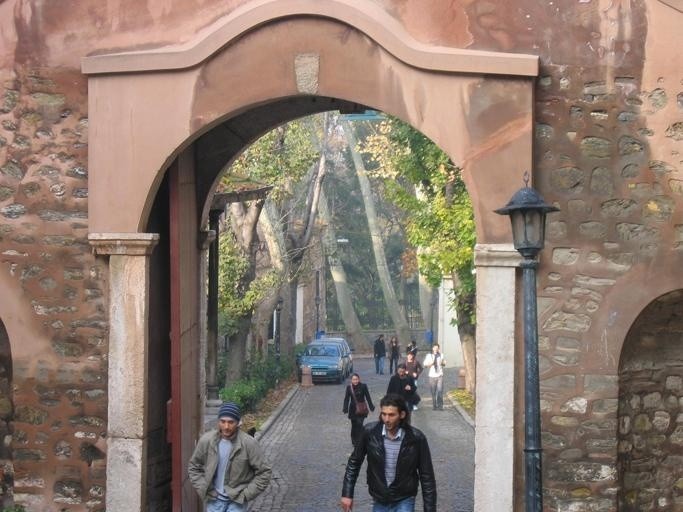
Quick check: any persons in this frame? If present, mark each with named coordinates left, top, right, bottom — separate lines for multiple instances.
left=405, top=341, right=418, bottom=357
left=187, top=401, right=273, bottom=512
left=340, top=392, right=438, bottom=512
left=403, top=351, right=423, bottom=411
left=386, top=363, right=417, bottom=426
left=423, top=343, right=446, bottom=411
left=387, top=336, right=401, bottom=375
left=342, top=373, right=375, bottom=445
left=373, top=334, right=386, bottom=376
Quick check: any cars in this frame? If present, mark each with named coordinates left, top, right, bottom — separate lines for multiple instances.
left=295, top=337, right=354, bottom=384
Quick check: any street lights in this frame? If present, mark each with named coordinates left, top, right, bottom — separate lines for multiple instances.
left=492, top=170, right=560, bottom=511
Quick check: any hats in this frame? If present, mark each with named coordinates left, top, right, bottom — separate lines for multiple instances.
left=218, top=402, right=241, bottom=420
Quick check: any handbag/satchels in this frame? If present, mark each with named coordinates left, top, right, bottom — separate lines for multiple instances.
left=356, top=402, right=369, bottom=415
left=407, top=392, right=420, bottom=404
